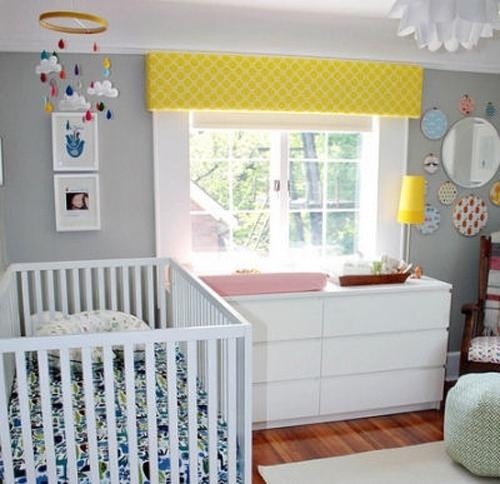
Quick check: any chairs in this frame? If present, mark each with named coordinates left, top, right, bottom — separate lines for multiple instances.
left=460, top=235, right=500, bottom=377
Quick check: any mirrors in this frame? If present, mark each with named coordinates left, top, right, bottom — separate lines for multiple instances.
left=441, top=117, right=500, bottom=189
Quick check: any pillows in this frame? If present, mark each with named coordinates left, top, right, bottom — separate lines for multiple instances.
left=30, top=309, right=154, bottom=371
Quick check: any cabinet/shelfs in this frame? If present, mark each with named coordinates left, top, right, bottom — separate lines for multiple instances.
left=217, top=274, right=452, bottom=433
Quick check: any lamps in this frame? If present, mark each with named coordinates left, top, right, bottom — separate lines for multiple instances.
left=399, top=174, right=426, bottom=266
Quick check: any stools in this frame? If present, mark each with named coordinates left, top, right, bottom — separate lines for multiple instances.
left=444, top=371, right=500, bottom=478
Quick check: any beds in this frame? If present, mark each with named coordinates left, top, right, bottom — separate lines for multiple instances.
left=0, top=260, right=255, bottom=484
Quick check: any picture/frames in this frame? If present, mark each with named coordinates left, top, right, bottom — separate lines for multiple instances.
left=52, top=112, right=101, bottom=233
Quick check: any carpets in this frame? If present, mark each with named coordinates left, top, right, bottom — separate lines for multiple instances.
left=258, top=440, right=500, bottom=484
